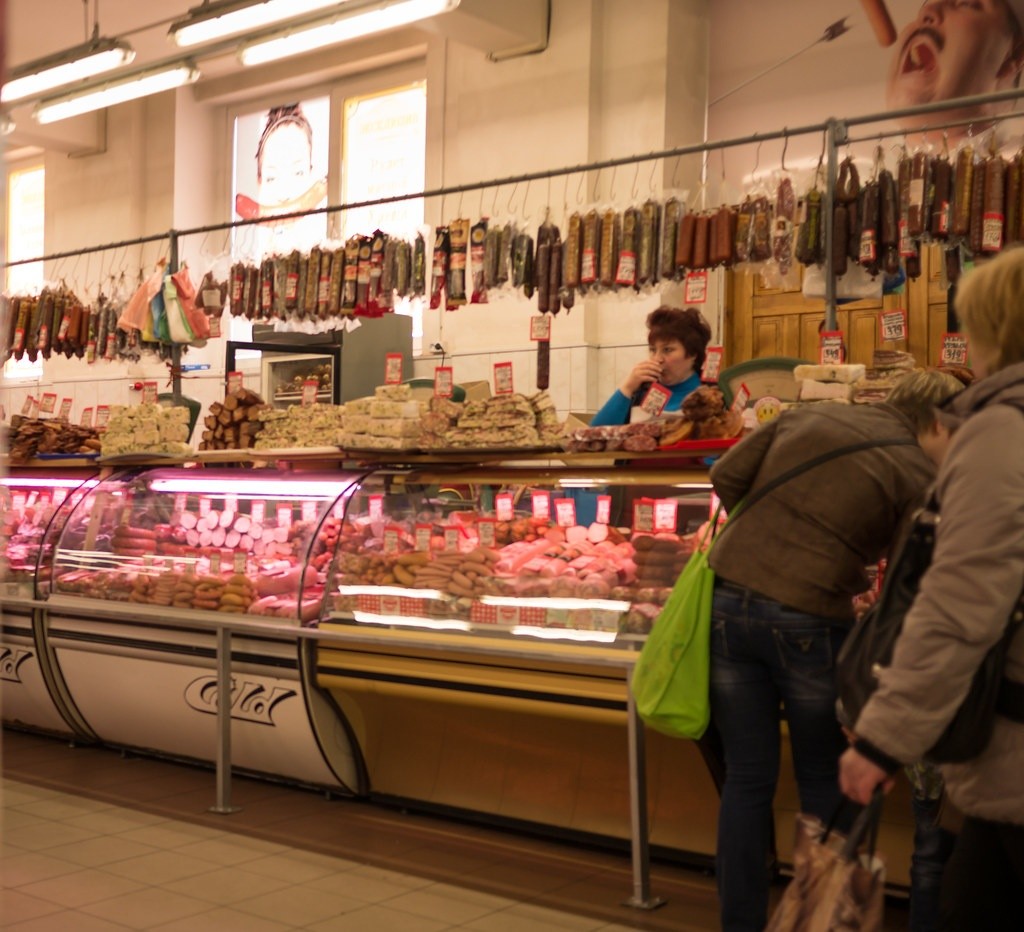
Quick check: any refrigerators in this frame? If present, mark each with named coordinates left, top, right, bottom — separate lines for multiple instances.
left=224, top=314, right=415, bottom=406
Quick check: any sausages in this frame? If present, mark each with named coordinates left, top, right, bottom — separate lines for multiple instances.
left=54, top=516, right=360, bottom=620
left=361, top=549, right=502, bottom=598
left=0, top=286, right=169, bottom=368
left=195, top=231, right=425, bottom=323
left=483, top=145, right=1023, bottom=318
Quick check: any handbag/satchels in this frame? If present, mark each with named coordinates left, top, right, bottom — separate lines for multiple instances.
left=832, top=403, right=1024, bottom=763
left=629, top=499, right=743, bottom=740
left=765, top=782, right=885, bottom=932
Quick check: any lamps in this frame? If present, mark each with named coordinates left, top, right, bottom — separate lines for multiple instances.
left=0, top=0, right=139, bottom=104
left=31, top=0, right=205, bottom=127
left=236, top=0, right=464, bottom=68
left=168, top=0, right=353, bottom=51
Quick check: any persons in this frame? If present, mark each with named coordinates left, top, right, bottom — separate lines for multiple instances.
left=829, top=246, right=1024, bottom=932
left=885, top=0, right=1024, bottom=148
left=258, top=104, right=313, bottom=206
left=590, top=307, right=714, bottom=531
left=706, top=369, right=966, bottom=932
